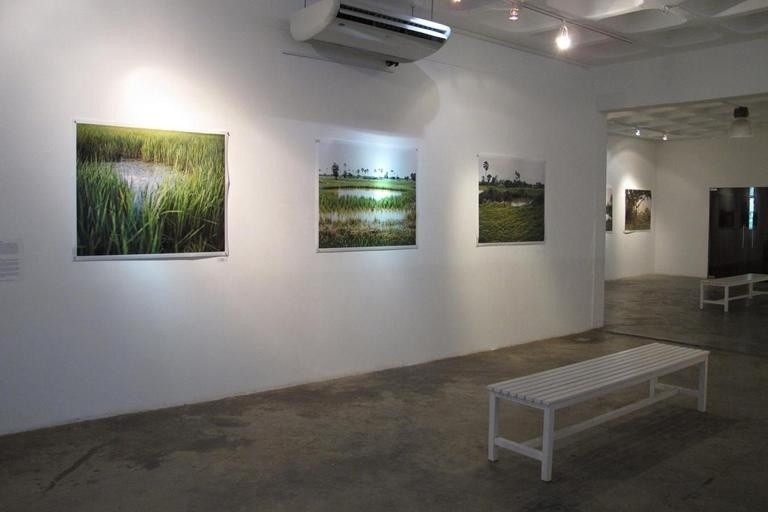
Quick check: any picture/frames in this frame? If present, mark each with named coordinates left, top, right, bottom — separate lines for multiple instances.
left=623, top=187, right=654, bottom=233
left=606, top=186, right=614, bottom=233
left=475, top=152, right=548, bottom=246
left=73, top=119, right=229, bottom=259
left=314, top=136, right=419, bottom=252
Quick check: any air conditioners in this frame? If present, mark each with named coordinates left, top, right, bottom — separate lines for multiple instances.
left=283, top=0, right=451, bottom=72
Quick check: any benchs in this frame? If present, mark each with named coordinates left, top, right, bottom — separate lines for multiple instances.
left=699, top=273, right=768, bottom=313
left=486, top=342, right=711, bottom=482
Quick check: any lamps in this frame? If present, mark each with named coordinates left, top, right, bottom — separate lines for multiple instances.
left=730, top=106, right=751, bottom=137
left=451, top=0, right=633, bottom=51
